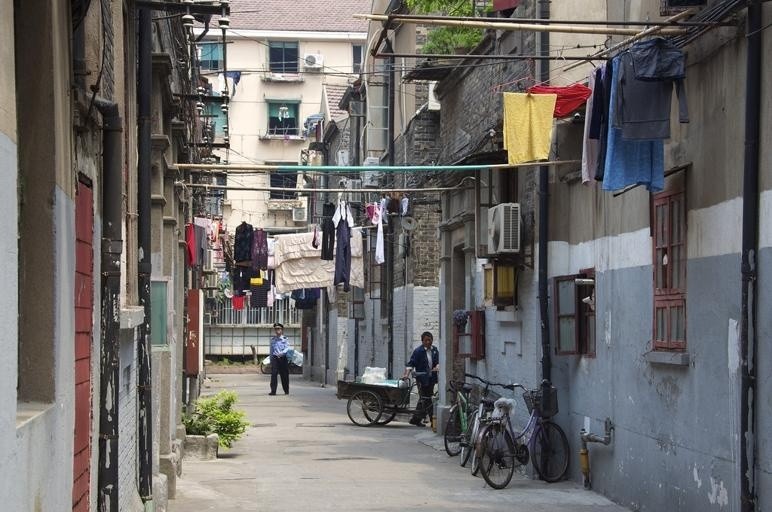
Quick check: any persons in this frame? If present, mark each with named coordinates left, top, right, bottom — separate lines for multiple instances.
left=268, top=323, right=290, bottom=396
left=404, top=331, right=440, bottom=429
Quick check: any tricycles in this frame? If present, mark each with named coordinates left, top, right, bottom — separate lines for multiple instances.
left=336, top=371, right=436, bottom=425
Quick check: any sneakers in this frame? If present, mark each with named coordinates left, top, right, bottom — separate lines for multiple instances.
left=408, top=419, right=427, bottom=428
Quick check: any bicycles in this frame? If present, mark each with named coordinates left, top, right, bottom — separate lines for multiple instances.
left=475, top=379, right=571, bottom=490
left=261, top=348, right=302, bottom=375
left=444, top=377, right=477, bottom=456
left=460, top=373, right=515, bottom=475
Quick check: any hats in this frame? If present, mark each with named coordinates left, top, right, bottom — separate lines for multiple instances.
left=272, top=322, right=284, bottom=329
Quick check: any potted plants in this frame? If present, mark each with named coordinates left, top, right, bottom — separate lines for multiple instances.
left=422, top=25, right=484, bottom=66
left=180, top=388, right=253, bottom=461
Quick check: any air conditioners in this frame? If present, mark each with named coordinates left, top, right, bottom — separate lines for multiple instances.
left=361, top=157, right=379, bottom=188
left=486, top=202, right=521, bottom=256
left=335, top=150, right=350, bottom=168
left=303, top=53, right=324, bottom=69
left=428, top=81, right=441, bottom=112
left=292, top=208, right=308, bottom=222
left=347, top=179, right=362, bottom=203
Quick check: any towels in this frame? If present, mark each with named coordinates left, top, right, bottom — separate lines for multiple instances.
left=503, top=92, right=557, bottom=167
left=232, top=295, right=244, bottom=310
